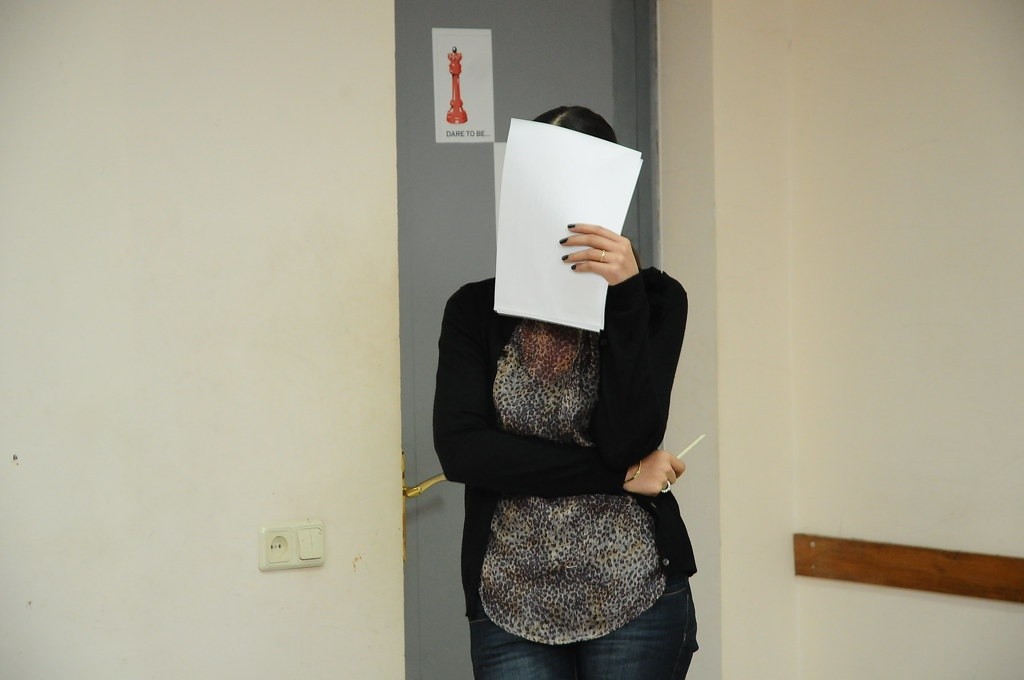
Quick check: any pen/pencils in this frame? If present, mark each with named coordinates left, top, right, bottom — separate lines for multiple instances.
left=677, top=433, right=707, bottom=460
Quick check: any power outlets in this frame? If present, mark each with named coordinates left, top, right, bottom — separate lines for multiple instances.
left=257, top=517, right=327, bottom=571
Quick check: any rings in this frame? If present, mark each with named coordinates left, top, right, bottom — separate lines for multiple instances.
left=599, top=250, right=605, bottom=261
left=661, top=481, right=670, bottom=493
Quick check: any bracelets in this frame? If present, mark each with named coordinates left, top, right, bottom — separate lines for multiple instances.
left=624, top=460, right=641, bottom=483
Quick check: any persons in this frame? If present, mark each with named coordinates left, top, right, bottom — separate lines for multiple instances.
left=432, top=104, right=700, bottom=680
